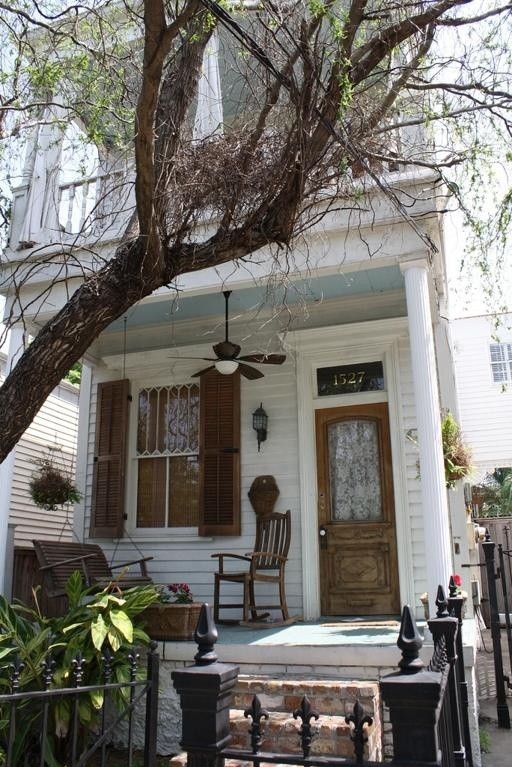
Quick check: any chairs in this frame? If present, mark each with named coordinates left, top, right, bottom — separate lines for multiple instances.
left=211, top=509, right=300, bottom=628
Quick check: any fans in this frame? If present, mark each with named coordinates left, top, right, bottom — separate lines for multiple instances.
left=165, top=291, right=287, bottom=380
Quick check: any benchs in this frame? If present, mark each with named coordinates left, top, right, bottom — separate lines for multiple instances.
left=31, top=539, right=153, bottom=600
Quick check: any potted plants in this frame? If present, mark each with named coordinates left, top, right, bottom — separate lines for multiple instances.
left=27, top=450, right=86, bottom=510
left=406, top=412, right=481, bottom=491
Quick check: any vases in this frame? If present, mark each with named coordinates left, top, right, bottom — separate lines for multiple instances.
left=133, top=602, right=206, bottom=640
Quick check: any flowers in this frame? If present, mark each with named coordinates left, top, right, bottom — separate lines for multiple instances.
left=156, top=583, right=195, bottom=603
left=452, top=575, right=463, bottom=593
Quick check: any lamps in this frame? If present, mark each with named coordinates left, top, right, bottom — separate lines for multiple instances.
left=477, top=526, right=486, bottom=542
left=252, top=402, right=268, bottom=451
